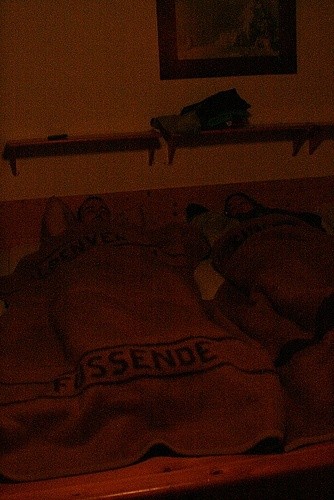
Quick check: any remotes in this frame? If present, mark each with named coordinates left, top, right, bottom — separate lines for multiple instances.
left=48, top=134, right=67, bottom=140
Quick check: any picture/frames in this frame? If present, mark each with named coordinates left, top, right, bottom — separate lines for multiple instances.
left=156, top=0, right=297, bottom=81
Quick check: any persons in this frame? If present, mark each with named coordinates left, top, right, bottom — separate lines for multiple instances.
left=37, top=193, right=147, bottom=262
left=223, top=192, right=334, bottom=240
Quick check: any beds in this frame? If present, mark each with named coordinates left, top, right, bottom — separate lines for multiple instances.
left=0, top=175, right=334, bottom=500
